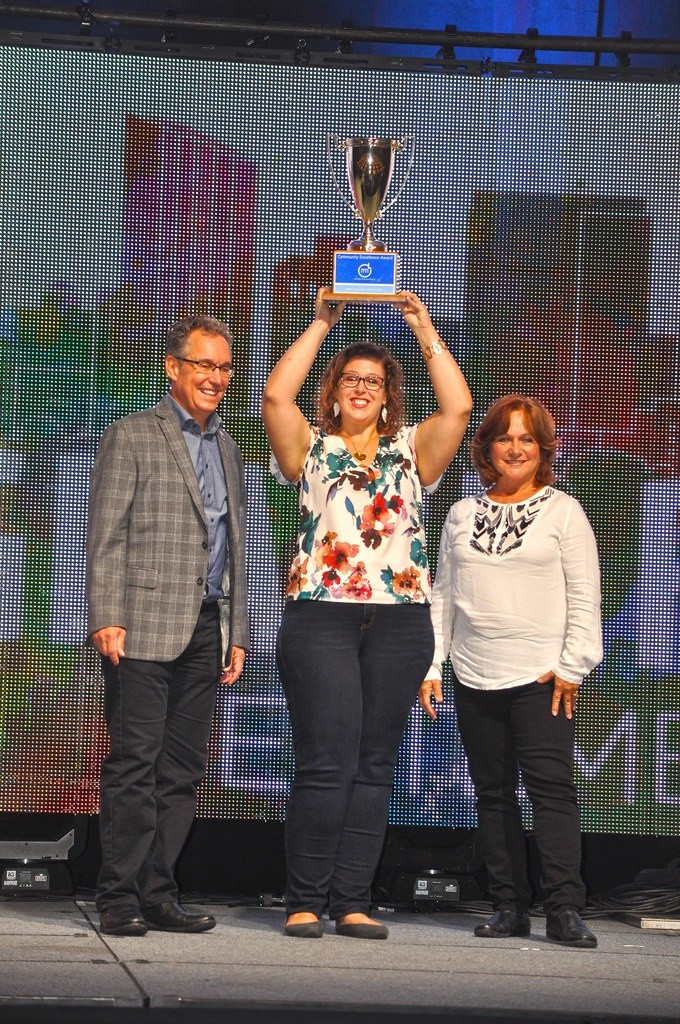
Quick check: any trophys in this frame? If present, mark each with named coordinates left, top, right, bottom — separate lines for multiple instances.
left=321, top=132, right=417, bottom=303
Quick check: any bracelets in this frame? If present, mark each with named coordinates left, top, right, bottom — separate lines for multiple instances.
left=422, top=340, right=449, bottom=362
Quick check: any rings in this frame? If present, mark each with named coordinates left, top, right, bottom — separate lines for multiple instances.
left=571, top=693, right=579, bottom=699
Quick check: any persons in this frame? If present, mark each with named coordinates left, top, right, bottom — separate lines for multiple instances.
left=419, top=395, right=607, bottom=952
left=82, top=309, right=253, bottom=938
left=261, top=289, right=481, bottom=942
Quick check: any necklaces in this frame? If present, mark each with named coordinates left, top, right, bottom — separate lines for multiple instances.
left=340, top=435, right=375, bottom=462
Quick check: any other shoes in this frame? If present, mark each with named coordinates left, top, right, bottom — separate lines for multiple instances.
left=335, top=923, right=389, bottom=939
left=286, top=916, right=323, bottom=936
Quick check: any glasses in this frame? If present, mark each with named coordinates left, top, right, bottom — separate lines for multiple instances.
left=174, top=356, right=235, bottom=379
left=338, top=373, right=387, bottom=391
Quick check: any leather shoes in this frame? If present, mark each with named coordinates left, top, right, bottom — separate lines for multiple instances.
left=546, top=910, right=598, bottom=948
left=100, top=904, right=147, bottom=936
left=474, top=910, right=531, bottom=937
left=143, top=901, right=216, bottom=932
